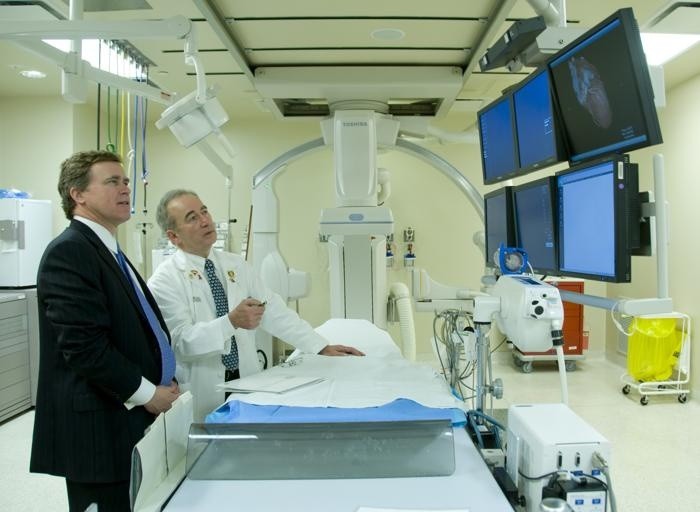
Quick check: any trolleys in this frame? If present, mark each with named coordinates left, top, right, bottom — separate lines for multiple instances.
left=620, top=311, right=692, bottom=406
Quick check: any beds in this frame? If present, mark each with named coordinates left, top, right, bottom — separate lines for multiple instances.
left=158, top=317, right=517, bottom=512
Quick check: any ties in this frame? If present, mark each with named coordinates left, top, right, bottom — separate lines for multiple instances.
left=114, top=242, right=175, bottom=387
left=205, top=259, right=238, bottom=373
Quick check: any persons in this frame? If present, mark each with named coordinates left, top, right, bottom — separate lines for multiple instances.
left=29, top=151, right=178, bottom=511
left=146, top=190, right=364, bottom=425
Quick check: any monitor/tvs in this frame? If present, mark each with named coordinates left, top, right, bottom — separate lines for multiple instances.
left=555, top=153, right=641, bottom=284
left=476, top=90, right=528, bottom=185
left=510, top=63, right=568, bottom=176
left=511, top=175, right=561, bottom=277
left=543, top=7, right=664, bottom=168
left=484, top=186, right=515, bottom=268
